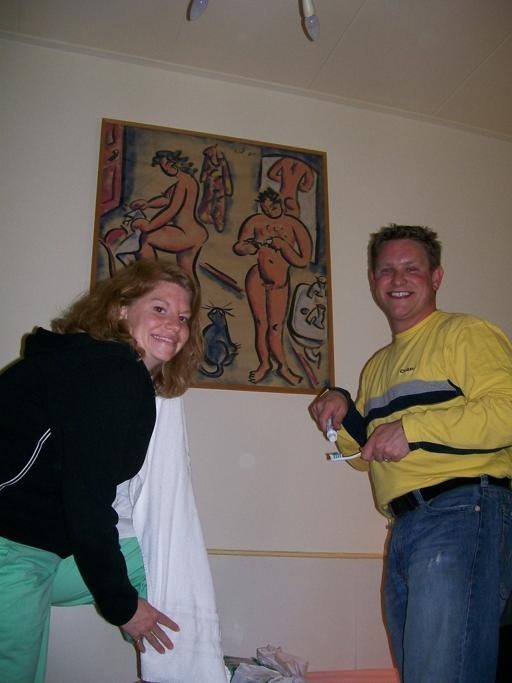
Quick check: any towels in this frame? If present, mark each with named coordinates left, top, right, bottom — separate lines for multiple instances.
left=111, top=390, right=232, bottom=683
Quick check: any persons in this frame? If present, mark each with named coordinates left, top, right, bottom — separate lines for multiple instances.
left=234, top=187, right=313, bottom=385
left=307, top=224, right=512, bottom=683
left=266, top=157, right=315, bottom=220
left=0, top=259, right=204, bottom=683
left=130, top=149, right=208, bottom=284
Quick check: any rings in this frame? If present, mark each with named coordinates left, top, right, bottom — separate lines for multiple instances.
left=134, top=637, right=141, bottom=644
left=383, top=457, right=392, bottom=462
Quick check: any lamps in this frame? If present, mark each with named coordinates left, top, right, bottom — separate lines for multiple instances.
left=302, top=1, right=320, bottom=42
left=189, top=0, right=208, bottom=20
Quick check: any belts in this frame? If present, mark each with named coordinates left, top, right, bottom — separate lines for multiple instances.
left=387, top=474, right=511, bottom=516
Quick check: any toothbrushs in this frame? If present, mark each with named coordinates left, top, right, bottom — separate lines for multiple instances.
left=328, top=451, right=363, bottom=462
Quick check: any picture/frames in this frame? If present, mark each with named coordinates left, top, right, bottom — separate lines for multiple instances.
left=91, top=118, right=337, bottom=395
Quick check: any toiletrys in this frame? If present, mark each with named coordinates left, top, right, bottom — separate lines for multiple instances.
left=327, top=415, right=337, bottom=443
